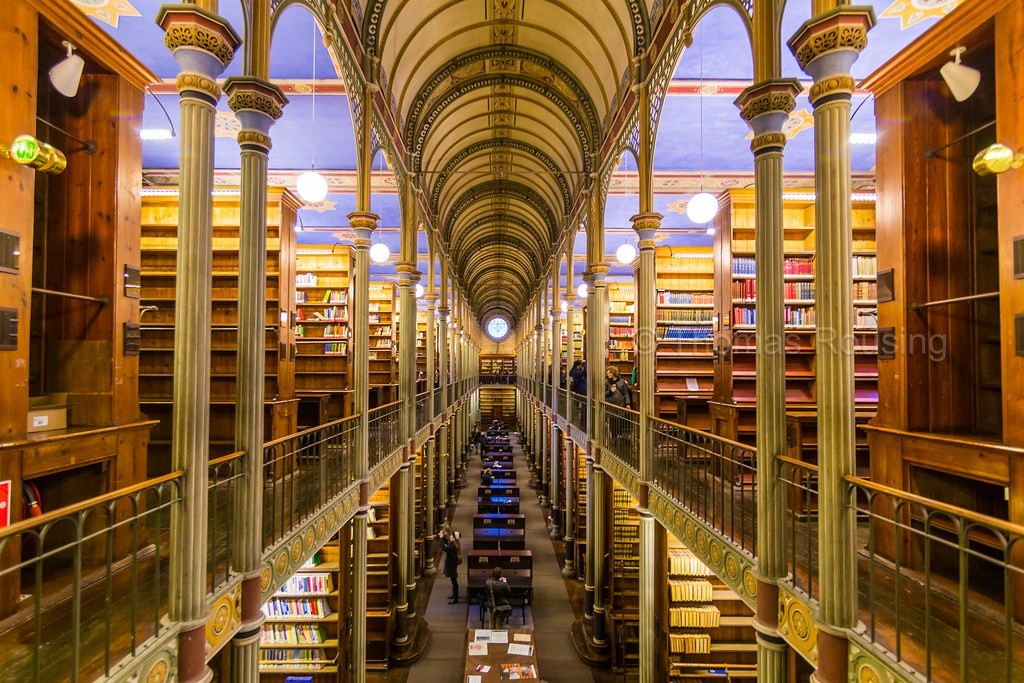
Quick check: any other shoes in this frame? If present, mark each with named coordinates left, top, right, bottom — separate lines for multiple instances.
left=448, top=600, right=459, bottom=604
left=447, top=595, right=454, bottom=599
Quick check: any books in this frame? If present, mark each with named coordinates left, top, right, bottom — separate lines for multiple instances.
left=323, top=290, right=345, bottom=304
left=609, top=301, right=635, bottom=361
left=578, top=450, right=720, bottom=676
left=296, top=306, right=348, bottom=355
left=852, top=256, right=878, bottom=328
left=783, top=259, right=816, bottom=326
left=369, top=287, right=423, bottom=360
left=296, top=273, right=317, bottom=287
left=541, top=331, right=567, bottom=363
left=573, top=325, right=582, bottom=357
left=731, top=256, right=757, bottom=325
left=657, top=290, right=714, bottom=338
left=259, top=557, right=334, bottom=669
left=296, top=291, right=306, bottom=304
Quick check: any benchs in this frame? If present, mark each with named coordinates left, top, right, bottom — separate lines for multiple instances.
left=476, top=590, right=530, bottom=629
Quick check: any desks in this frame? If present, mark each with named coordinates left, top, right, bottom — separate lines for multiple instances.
left=464, top=627, right=540, bottom=683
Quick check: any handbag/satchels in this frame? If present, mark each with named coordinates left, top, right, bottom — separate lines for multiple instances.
left=570, top=381, right=575, bottom=391
left=496, top=605, right=513, bottom=618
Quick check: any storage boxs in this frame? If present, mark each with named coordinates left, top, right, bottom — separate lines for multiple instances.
left=26, top=392, right=80, bottom=433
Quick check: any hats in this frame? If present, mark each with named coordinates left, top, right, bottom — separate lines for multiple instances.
left=453, top=532, right=461, bottom=539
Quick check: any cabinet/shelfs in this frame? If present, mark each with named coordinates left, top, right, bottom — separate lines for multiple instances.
left=0, top=0, right=1024, bottom=683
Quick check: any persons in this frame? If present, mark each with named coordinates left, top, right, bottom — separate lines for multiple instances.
left=542, top=360, right=587, bottom=430
left=484, top=567, right=511, bottom=629
left=478, top=421, right=501, bottom=446
left=604, top=366, right=630, bottom=444
left=481, top=467, right=493, bottom=486
left=438, top=530, right=461, bottom=605
left=434, top=368, right=439, bottom=388
left=472, top=428, right=481, bottom=454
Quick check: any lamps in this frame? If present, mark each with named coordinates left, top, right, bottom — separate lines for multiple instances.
left=615, top=150, right=636, bottom=264
left=685, top=19, right=719, bottom=225
left=972, top=142, right=1024, bottom=177
left=1, top=133, right=68, bottom=176
left=370, top=150, right=391, bottom=264
left=294, top=16, right=330, bottom=205
left=48, top=40, right=86, bottom=98
left=939, top=46, right=981, bottom=103
left=577, top=226, right=589, bottom=298
